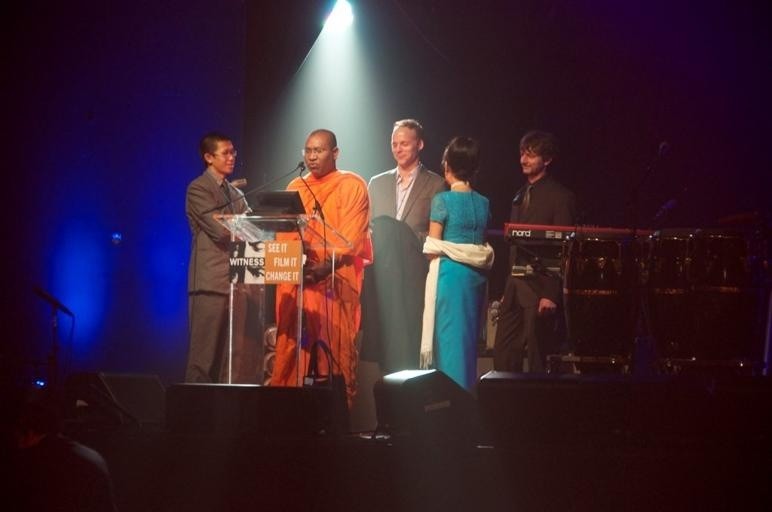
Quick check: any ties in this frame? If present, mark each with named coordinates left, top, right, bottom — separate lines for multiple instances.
left=518, top=185, right=531, bottom=215
left=221, top=181, right=230, bottom=200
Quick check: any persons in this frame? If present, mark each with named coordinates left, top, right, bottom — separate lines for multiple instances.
left=356, top=117, right=449, bottom=381
left=183, top=132, right=255, bottom=384
left=267, top=129, right=376, bottom=411
left=418, top=132, right=496, bottom=394
left=490, top=124, right=579, bottom=376
left=1, top=385, right=119, bottom=512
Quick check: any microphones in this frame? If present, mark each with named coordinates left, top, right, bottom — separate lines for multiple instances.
left=299, top=167, right=325, bottom=219
left=202, top=162, right=304, bottom=214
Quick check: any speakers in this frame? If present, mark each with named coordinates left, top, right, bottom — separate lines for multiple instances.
left=167, top=381, right=337, bottom=446
left=64, top=370, right=167, bottom=428
left=478, top=370, right=629, bottom=450
left=373, top=367, right=478, bottom=445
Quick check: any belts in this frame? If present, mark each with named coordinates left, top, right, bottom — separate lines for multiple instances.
left=512, top=263, right=528, bottom=277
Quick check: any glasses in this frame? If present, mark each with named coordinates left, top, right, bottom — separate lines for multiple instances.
left=213, top=149, right=237, bottom=158
left=302, top=149, right=329, bottom=156
